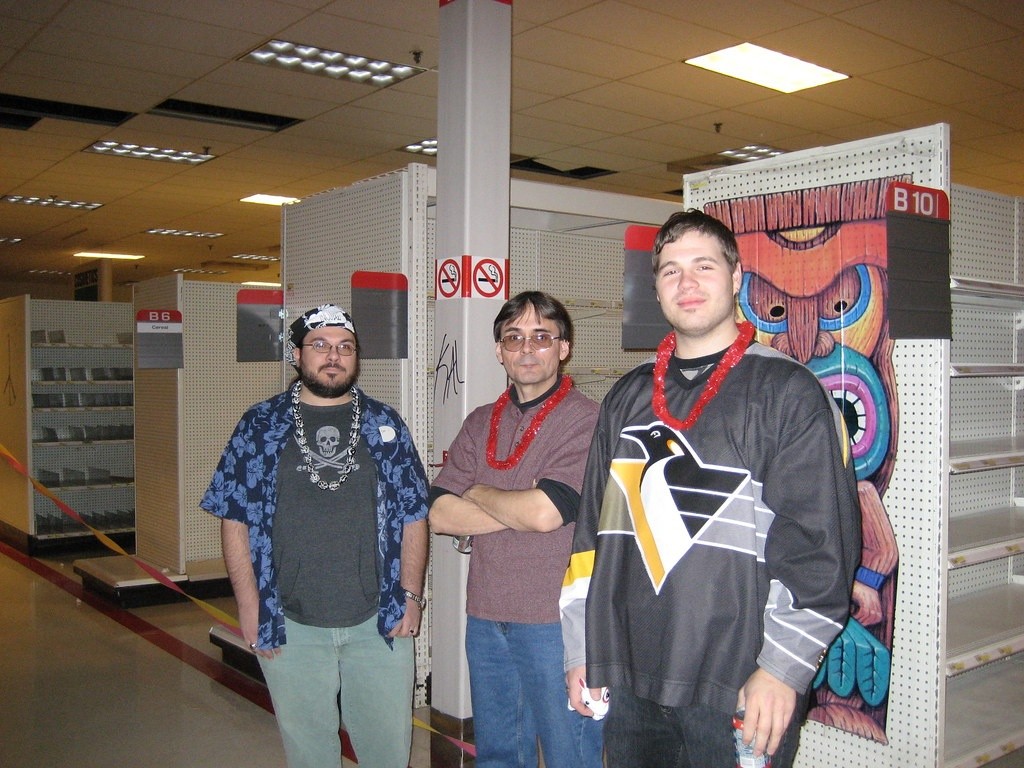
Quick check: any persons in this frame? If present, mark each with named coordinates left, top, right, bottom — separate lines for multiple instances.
left=559, top=210, right=862, bottom=768
left=427, top=291, right=604, bottom=768
left=197, top=304, right=430, bottom=768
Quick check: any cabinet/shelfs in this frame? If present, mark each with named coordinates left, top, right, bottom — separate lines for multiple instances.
left=1, top=295, right=135, bottom=539
left=417, top=163, right=678, bottom=409
left=684, top=123, right=1022, bottom=768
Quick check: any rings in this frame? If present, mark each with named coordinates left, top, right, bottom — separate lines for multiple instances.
left=250, top=643, right=257, bottom=650
left=409, top=629, right=414, bottom=633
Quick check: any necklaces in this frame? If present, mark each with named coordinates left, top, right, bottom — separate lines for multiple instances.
left=486, top=373, right=573, bottom=470
left=651, top=321, right=755, bottom=431
left=291, top=380, right=361, bottom=491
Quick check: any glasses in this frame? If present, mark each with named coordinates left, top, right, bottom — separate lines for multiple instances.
left=499, top=334, right=562, bottom=353
left=300, top=341, right=358, bottom=356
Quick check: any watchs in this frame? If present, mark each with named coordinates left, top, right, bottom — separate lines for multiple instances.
left=405, top=589, right=426, bottom=610
